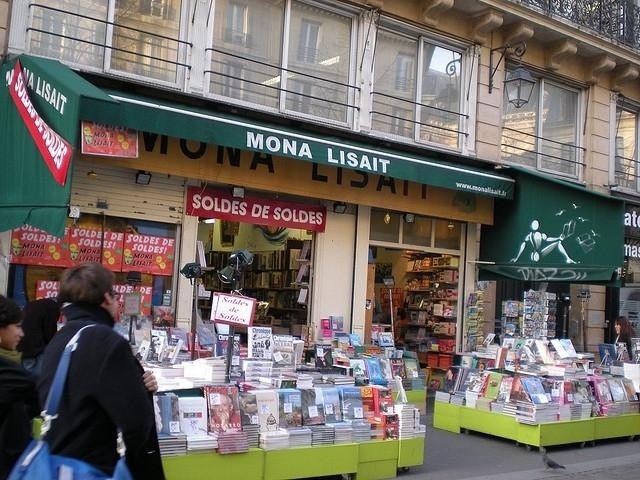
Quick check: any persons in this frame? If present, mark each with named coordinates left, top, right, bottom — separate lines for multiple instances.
left=18, top=296, right=60, bottom=411
left=38, top=261, right=168, bottom=480
left=1, top=293, right=42, bottom=480
left=612, top=315, right=638, bottom=360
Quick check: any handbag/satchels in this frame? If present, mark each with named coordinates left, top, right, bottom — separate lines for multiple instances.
left=9, top=440, right=132, bottom=480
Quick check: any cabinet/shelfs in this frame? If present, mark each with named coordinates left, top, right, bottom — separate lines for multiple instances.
left=404, top=253, right=459, bottom=355
left=197, top=251, right=308, bottom=328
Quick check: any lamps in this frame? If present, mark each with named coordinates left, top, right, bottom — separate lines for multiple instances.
left=232, top=186, right=245, bottom=198
left=135, top=170, right=152, bottom=185
left=488, top=40, right=536, bottom=108
left=435, top=56, right=461, bottom=122
left=333, top=200, right=348, bottom=214
left=403, top=213, right=416, bottom=223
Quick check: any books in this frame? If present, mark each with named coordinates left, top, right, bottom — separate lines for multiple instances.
left=111, top=240, right=461, bottom=456
left=462, top=333, right=640, bottom=426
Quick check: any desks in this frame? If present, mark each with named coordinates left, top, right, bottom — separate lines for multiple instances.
left=433, top=400, right=640, bottom=453
left=161, top=437, right=425, bottom=480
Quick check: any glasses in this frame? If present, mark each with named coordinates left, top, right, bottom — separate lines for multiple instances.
left=111, top=290, right=122, bottom=301
